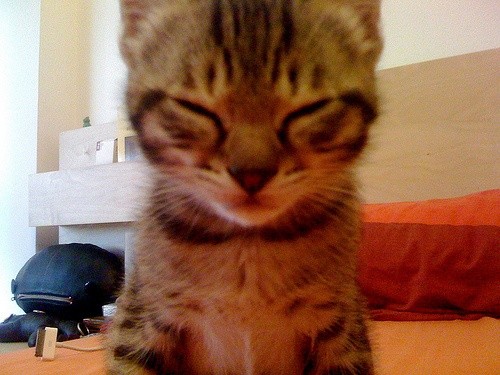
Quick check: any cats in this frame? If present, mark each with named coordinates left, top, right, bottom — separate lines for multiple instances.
left=102, top=1, right=383, bottom=375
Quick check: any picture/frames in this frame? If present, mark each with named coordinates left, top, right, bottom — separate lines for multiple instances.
left=117, top=105, right=148, bottom=162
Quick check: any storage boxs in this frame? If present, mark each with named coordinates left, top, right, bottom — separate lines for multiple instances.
left=95, top=138, right=116, bottom=165
